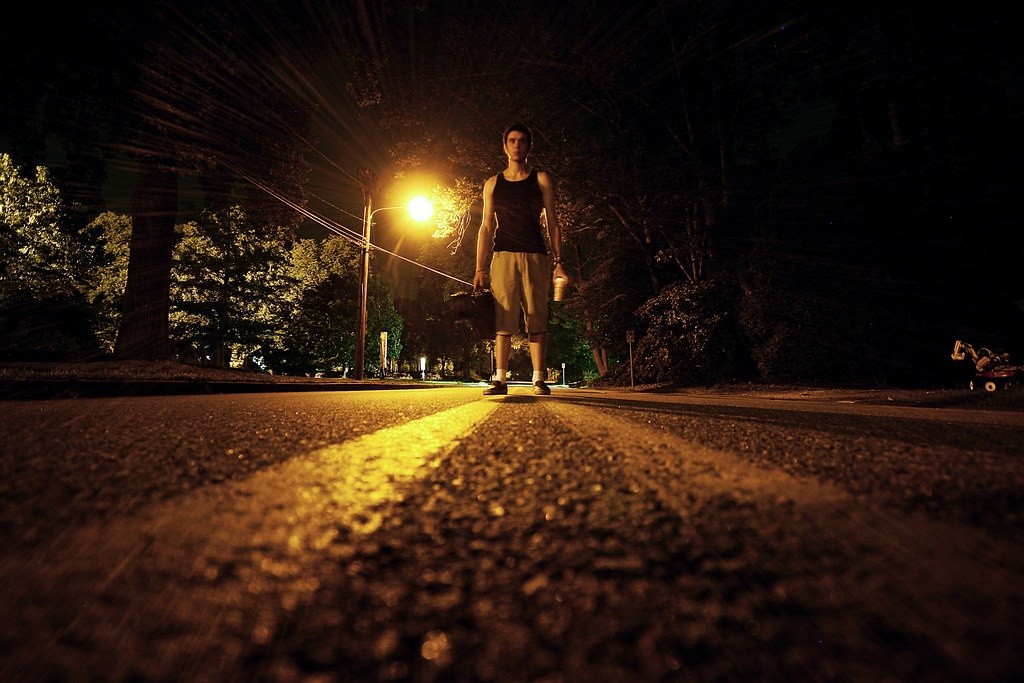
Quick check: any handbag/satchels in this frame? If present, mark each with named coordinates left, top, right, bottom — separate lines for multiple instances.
left=447, top=284, right=497, bottom=340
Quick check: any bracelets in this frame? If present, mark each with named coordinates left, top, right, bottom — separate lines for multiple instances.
left=475, top=269, right=484, bottom=273
left=554, top=260, right=563, bottom=266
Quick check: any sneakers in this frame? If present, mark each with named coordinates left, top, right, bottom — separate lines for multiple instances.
left=483, top=380, right=508, bottom=395
left=532, top=380, right=551, bottom=395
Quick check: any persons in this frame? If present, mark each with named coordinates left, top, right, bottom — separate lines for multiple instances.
left=473, top=123, right=574, bottom=395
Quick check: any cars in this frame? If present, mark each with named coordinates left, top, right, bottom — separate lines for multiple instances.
left=427, top=375, right=443, bottom=380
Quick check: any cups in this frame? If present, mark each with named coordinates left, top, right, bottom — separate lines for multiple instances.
left=553, top=278, right=567, bottom=302
